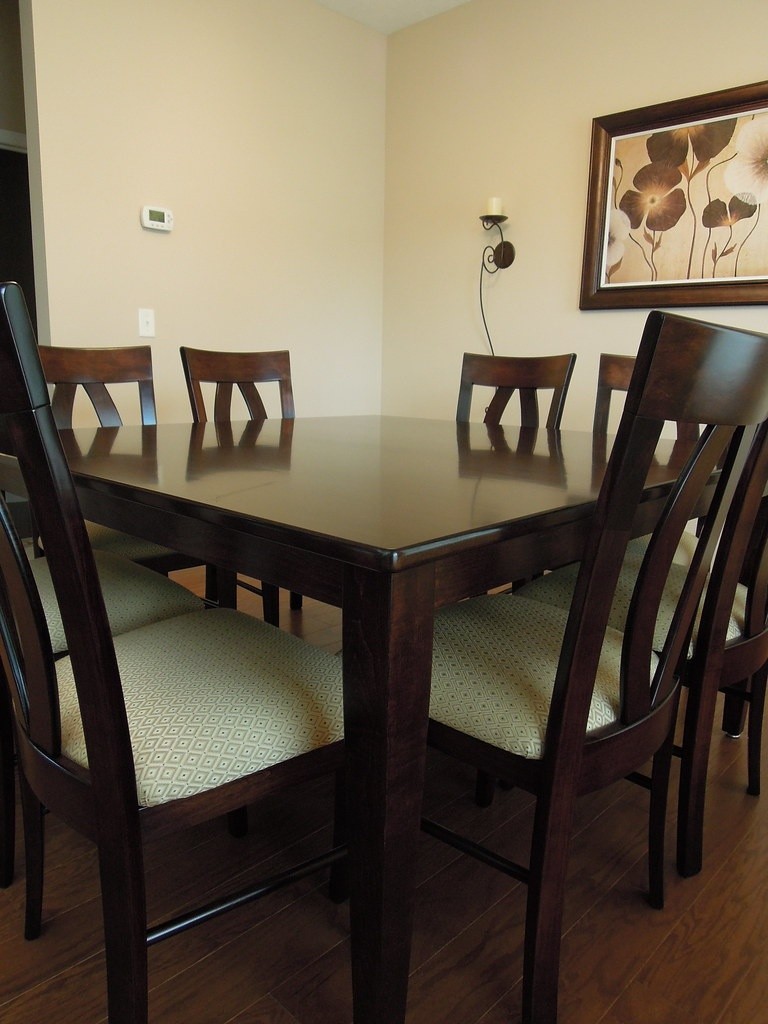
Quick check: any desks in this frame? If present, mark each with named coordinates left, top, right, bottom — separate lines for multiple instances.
left=0, top=412, right=767, bottom=1024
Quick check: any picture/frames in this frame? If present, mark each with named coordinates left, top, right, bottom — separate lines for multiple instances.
left=579, top=79, right=768, bottom=311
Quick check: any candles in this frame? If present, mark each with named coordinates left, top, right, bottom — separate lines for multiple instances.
left=485, top=197, right=502, bottom=215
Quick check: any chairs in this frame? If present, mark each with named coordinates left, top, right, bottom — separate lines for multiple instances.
left=0, top=277, right=768, bottom=1024
